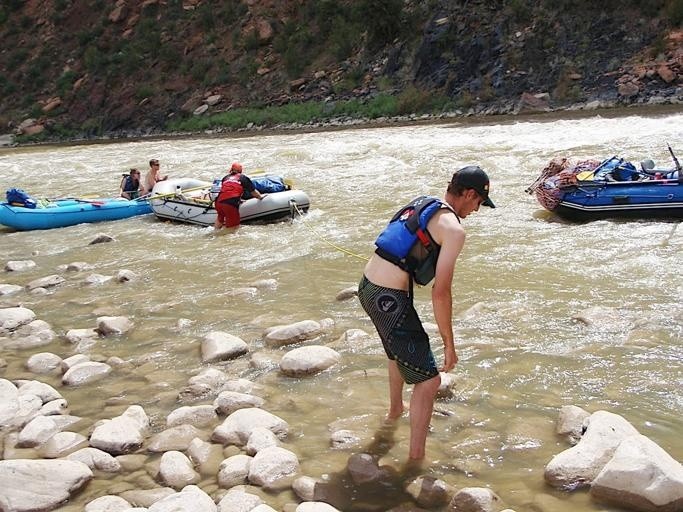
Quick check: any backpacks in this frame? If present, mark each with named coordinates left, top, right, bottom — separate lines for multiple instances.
left=7, top=187, right=37, bottom=209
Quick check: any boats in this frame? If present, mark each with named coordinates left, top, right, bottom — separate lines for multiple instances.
left=0, top=195, right=155, bottom=231
left=537, top=161, right=683, bottom=226
left=150, top=174, right=312, bottom=230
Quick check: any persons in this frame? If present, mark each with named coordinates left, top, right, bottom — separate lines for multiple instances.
left=356, top=166, right=496, bottom=460
left=144, top=159, right=168, bottom=192
left=119, top=168, right=146, bottom=200
left=213, top=162, right=266, bottom=228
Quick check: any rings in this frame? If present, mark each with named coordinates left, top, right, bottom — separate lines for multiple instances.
left=452, top=362, right=456, bottom=366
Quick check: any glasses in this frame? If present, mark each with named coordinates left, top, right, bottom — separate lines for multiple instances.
left=134, top=172, right=141, bottom=174
left=153, top=164, right=159, bottom=166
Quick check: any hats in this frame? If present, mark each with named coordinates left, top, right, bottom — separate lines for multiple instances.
left=229, top=163, right=242, bottom=173
left=451, top=166, right=496, bottom=209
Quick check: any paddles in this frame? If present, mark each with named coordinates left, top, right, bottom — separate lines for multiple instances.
left=75, top=200, right=104, bottom=207
left=576, top=155, right=617, bottom=181
left=145, top=169, right=266, bottom=201
